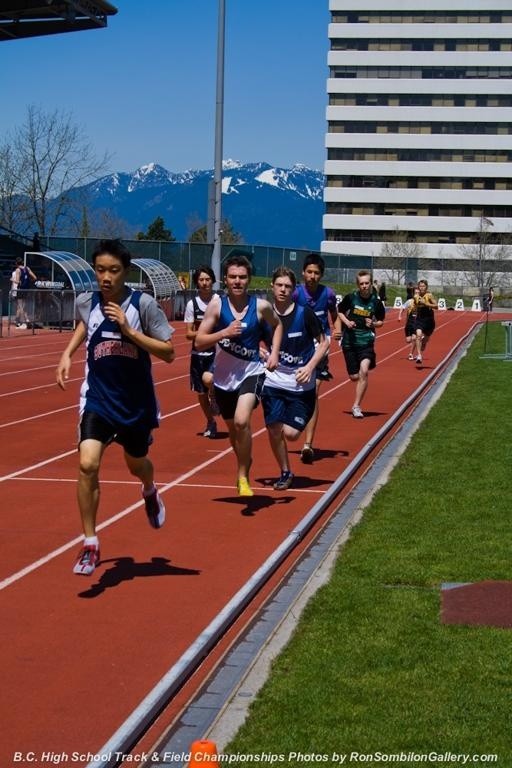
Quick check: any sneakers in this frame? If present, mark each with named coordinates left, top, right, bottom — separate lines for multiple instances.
left=143, top=489, right=166, bottom=528
left=205, top=420, right=217, bottom=438
left=299, top=443, right=314, bottom=463
left=74, top=545, right=101, bottom=576
left=413, top=354, right=417, bottom=359
left=274, top=471, right=293, bottom=489
left=237, top=477, right=253, bottom=497
left=408, top=353, right=413, bottom=360
left=415, top=355, right=422, bottom=363
left=352, top=405, right=363, bottom=417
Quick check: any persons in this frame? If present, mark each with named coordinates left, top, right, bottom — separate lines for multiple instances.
left=194, top=255, right=284, bottom=498
left=183, top=265, right=218, bottom=437
left=290, top=254, right=343, bottom=464
left=486, top=286, right=494, bottom=312
left=259, top=266, right=329, bottom=491
left=413, top=280, right=438, bottom=364
left=379, top=282, right=387, bottom=308
left=373, top=280, right=378, bottom=290
left=55, top=238, right=177, bottom=577
left=336, top=271, right=386, bottom=419
left=406, top=282, right=414, bottom=299
left=9, top=257, right=38, bottom=330
left=398, top=287, right=421, bottom=360
left=178, top=275, right=186, bottom=290
